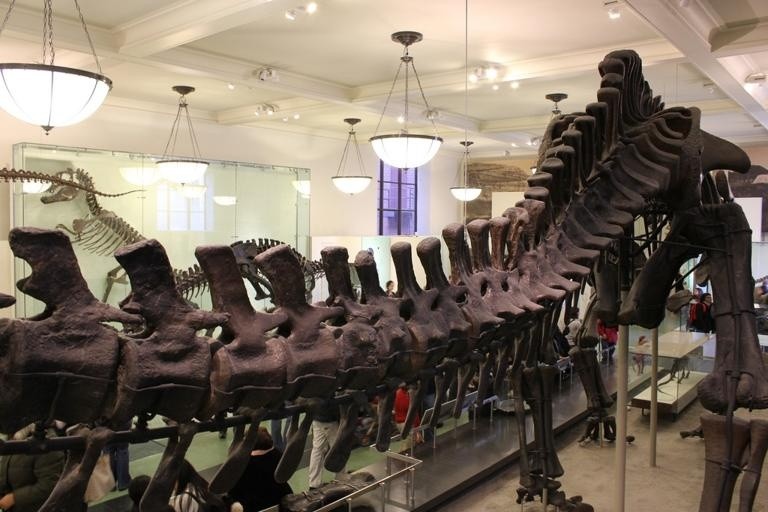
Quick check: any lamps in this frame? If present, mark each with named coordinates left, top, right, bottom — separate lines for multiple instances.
left=120, top=152, right=156, bottom=187
left=450, top=141, right=483, bottom=201
left=330, top=118, right=372, bottom=195
left=156, top=86, right=209, bottom=187
left=368, top=32, right=443, bottom=171
left=0, top=0, right=111, bottom=135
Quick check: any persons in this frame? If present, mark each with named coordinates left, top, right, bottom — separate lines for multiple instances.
left=562, top=307, right=583, bottom=347
left=633, top=335, right=650, bottom=376
left=359, top=322, right=570, bottom=434
left=168, top=459, right=210, bottom=512
left=689, top=293, right=715, bottom=333
left=127, top=473, right=175, bottom=511
left=385, top=280, right=396, bottom=297
left=107, top=417, right=132, bottom=491
left=228, top=426, right=293, bottom=511
left=597, top=320, right=619, bottom=364
left=0, top=429, right=74, bottom=511
left=307, top=404, right=350, bottom=491
left=690, top=287, right=703, bottom=304
left=753, top=285, right=768, bottom=309
